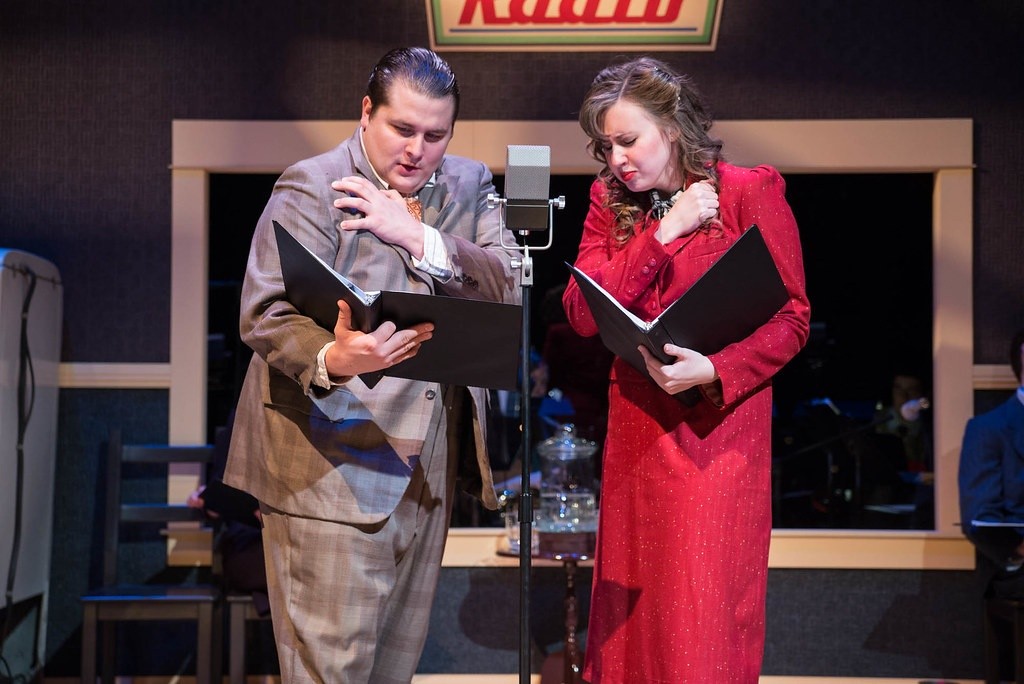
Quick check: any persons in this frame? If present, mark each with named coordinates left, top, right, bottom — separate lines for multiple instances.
left=958, top=328, right=1024, bottom=583
left=561, top=58, right=811, bottom=684
left=224, top=47, right=525, bottom=684
left=875, top=367, right=935, bottom=485
left=493, top=351, right=574, bottom=498
left=193, top=414, right=265, bottom=597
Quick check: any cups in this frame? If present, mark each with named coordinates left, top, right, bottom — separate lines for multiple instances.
left=506, top=503, right=520, bottom=554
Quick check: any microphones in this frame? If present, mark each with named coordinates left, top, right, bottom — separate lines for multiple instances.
left=504, top=145, right=550, bottom=237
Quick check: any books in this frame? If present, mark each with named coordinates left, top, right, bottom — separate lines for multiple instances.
left=270, top=219, right=522, bottom=391
left=564, top=223, right=790, bottom=407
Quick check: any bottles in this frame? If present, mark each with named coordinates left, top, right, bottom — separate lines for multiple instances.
left=534, top=423, right=599, bottom=562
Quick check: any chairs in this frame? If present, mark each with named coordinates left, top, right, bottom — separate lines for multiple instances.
left=224, top=428, right=272, bottom=684
left=79, top=428, right=224, bottom=684
left=966, top=521, right=1024, bottom=684
